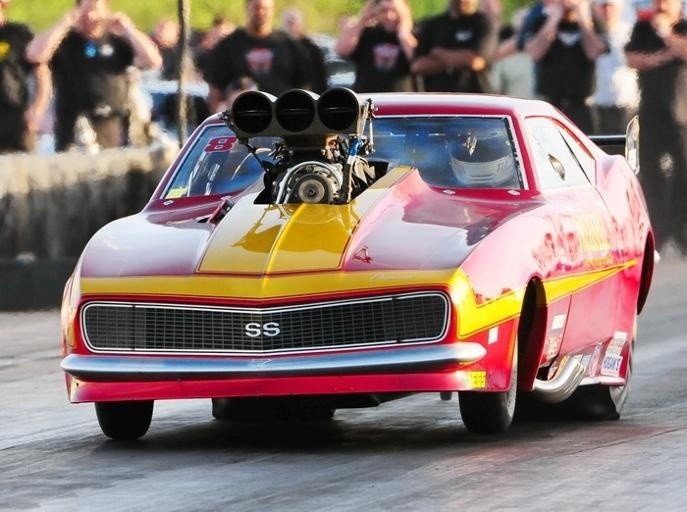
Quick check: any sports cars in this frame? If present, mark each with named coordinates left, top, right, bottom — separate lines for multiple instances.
left=61, top=92, right=655, bottom=439
